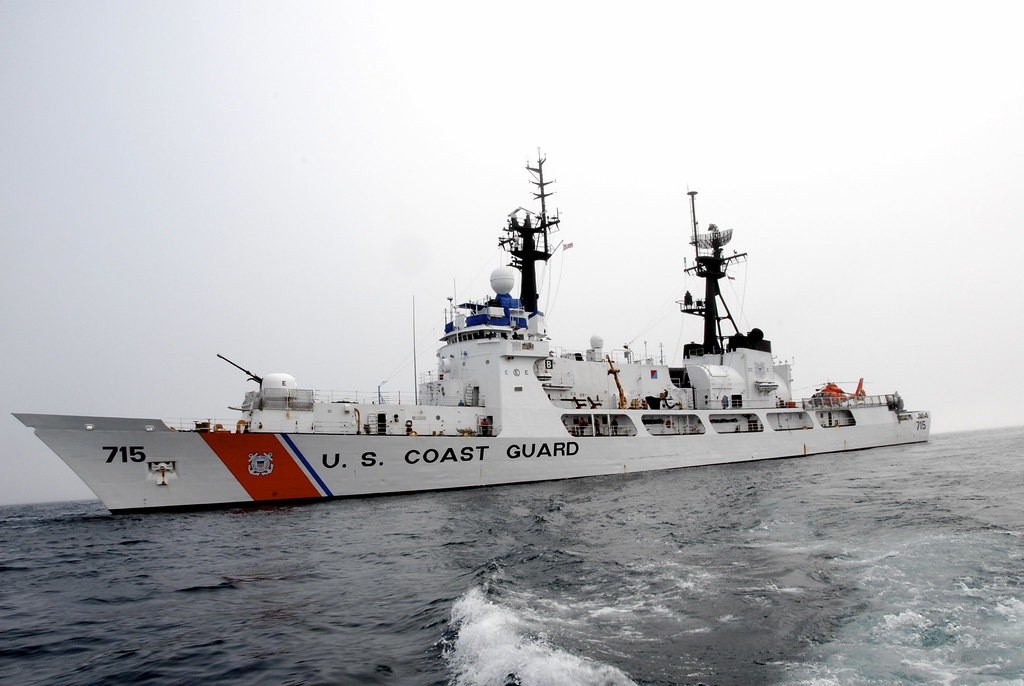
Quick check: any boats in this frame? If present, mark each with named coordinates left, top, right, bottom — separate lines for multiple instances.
left=9, top=148, right=932, bottom=517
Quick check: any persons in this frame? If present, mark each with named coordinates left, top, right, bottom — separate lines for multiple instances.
left=244, top=426, right=248, bottom=432
left=612, top=419, right=618, bottom=434
left=488, top=330, right=493, bottom=337
left=481, top=419, right=489, bottom=436
left=579, top=417, right=586, bottom=435
left=775, top=396, right=784, bottom=407
left=512, top=331, right=520, bottom=340
left=458, top=400, right=464, bottom=406
left=563, top=418, right=568, bottom=426
left=594, top=418, right=600, bottom=434
left=736, top=425, right=741, bottom=431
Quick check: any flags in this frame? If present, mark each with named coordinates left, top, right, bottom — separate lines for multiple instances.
left=564, top=243, right=573, bottom=250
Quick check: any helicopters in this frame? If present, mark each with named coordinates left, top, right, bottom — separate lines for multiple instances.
left=809, top=378, right=873, bottom=406
